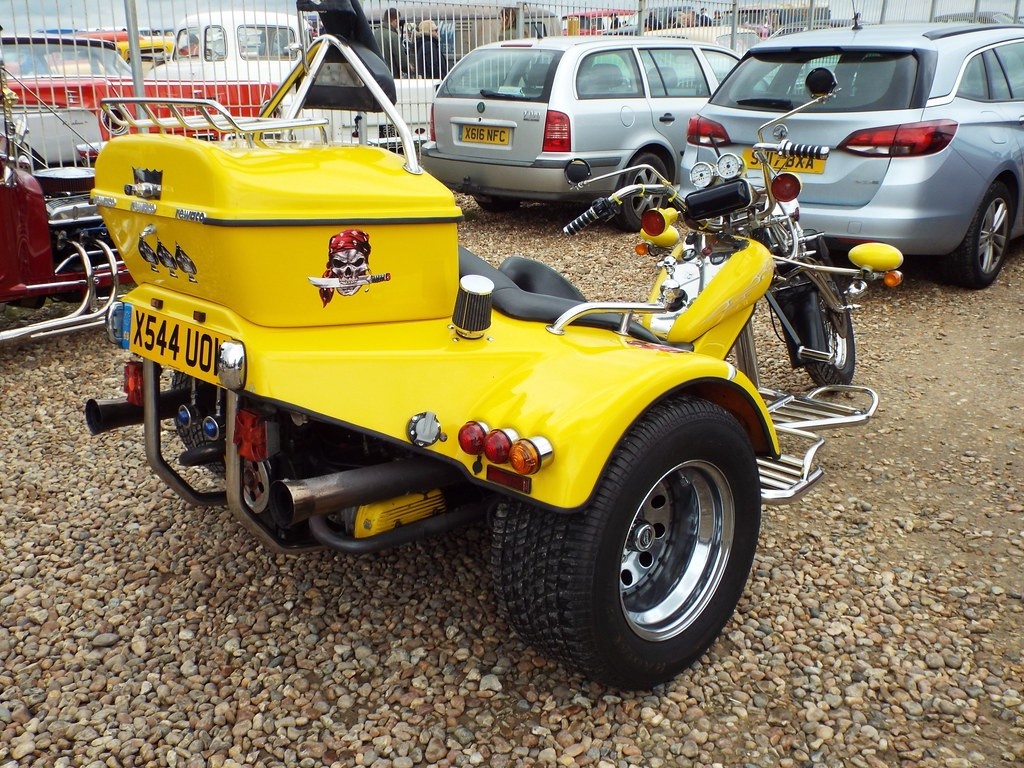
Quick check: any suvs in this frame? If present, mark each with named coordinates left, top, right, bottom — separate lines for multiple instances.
left=677, top=18, right=1024, bottom=290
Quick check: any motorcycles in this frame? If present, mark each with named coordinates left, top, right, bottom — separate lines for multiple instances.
left=83, top=34, right=912, bottom=696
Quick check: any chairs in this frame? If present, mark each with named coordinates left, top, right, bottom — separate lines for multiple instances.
left=844, top=58, right=892, bottom=106
left=259, top=41, right=281, bottom=59
left=522, top=62, right=552, bottom=97
left=647, top=66, right=678, bottom=98
left=582, top=63, right=622, bottom=97
left=968, top=54, right=1010, bottom=95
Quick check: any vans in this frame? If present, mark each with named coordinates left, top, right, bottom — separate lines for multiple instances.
left=357, top=6, right=560, bottom=78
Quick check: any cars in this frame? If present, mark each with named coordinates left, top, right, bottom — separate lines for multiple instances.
left=418, top=18, right=866, bottom=233
left=0, top=10, right=229, bottom=344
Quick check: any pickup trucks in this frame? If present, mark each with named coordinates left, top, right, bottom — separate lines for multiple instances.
left=144, top=9, right=441, bottom=146
